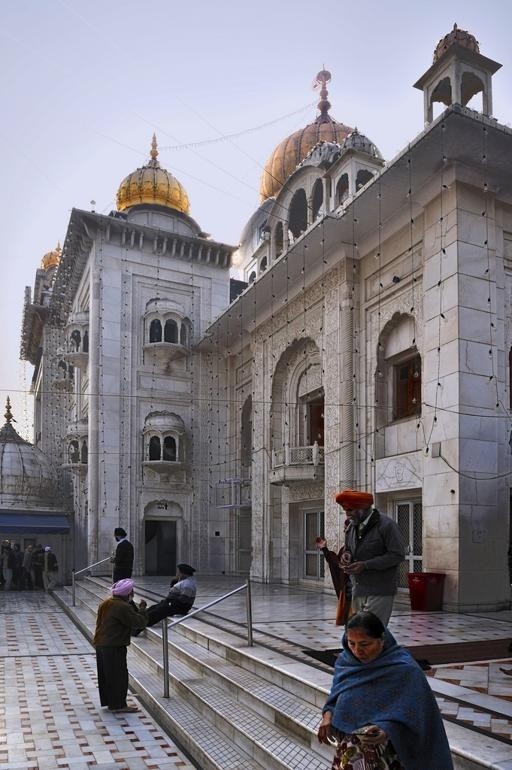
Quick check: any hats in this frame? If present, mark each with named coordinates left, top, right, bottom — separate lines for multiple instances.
left=45, top=547, right=51, bottom=551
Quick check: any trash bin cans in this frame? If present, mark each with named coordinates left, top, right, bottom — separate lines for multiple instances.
left=407, top=572, right=446, bottom=611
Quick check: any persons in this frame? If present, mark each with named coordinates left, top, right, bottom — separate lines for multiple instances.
left=316, top=609, right=458, bottom=769
left=130, top=563, right=197, bottom=638
left=93, top=576, right=150, bottom=715
left=332, top=485, right=406, bottom=634
left=1, top=539, right=64, bottom=594
left=314, top=518, right=364, bottom=627
left=111, top=527, right=135, bottom=583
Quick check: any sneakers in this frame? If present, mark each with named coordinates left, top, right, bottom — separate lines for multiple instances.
left=108, top=704, right=138, bottom=714
